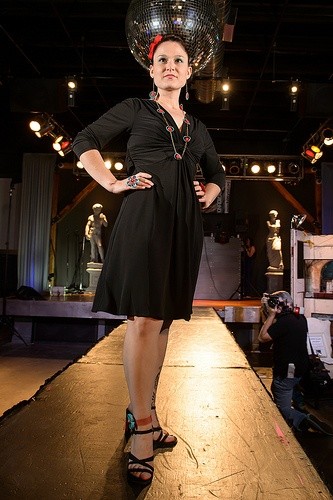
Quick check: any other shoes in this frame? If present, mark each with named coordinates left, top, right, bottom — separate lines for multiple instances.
left=299, top=412, right=329, bottom=435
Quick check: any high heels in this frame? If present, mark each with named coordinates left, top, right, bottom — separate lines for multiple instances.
left=122, top=406, right=178, bottom=488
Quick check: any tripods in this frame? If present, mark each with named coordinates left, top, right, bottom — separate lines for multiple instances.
left=228, top=250, right=262, bottom=300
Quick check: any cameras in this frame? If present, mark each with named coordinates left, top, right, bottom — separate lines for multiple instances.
left=265, top=295, right=279, bottom=309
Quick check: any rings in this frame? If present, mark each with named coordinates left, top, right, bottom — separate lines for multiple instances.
left=199, top=182, right=207, bottom=192
left=126, top=175, right=140, bottom=188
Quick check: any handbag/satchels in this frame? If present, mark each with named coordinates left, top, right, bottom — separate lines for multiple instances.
left=300, top=354, right=332, bottom=394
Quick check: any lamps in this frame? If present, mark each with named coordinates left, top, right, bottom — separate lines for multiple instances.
left=289, top=81, right=302, bottom=111
left=301, top=126, right=333, bottom=164
left=29, top=111, right=74, bottom=157
left=220, top=80, right=231, bottom=111
left=68, top=78, right=79, bottom=107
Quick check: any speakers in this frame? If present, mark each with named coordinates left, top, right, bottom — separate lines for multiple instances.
left=16, top=285, right=41, bottom=300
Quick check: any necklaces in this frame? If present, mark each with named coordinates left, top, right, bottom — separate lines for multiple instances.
left=152, top=94, right=192, bottom=160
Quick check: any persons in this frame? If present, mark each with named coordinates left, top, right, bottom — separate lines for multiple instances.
left=265, top=210, right=285, bottom=271
left=85, top=203, right=107, bottom=263
left=241, top=236, right=255, bottom=296
left=73, top=34, right=226, bottom=486
left=258, top=291, right=333, bottom=436
